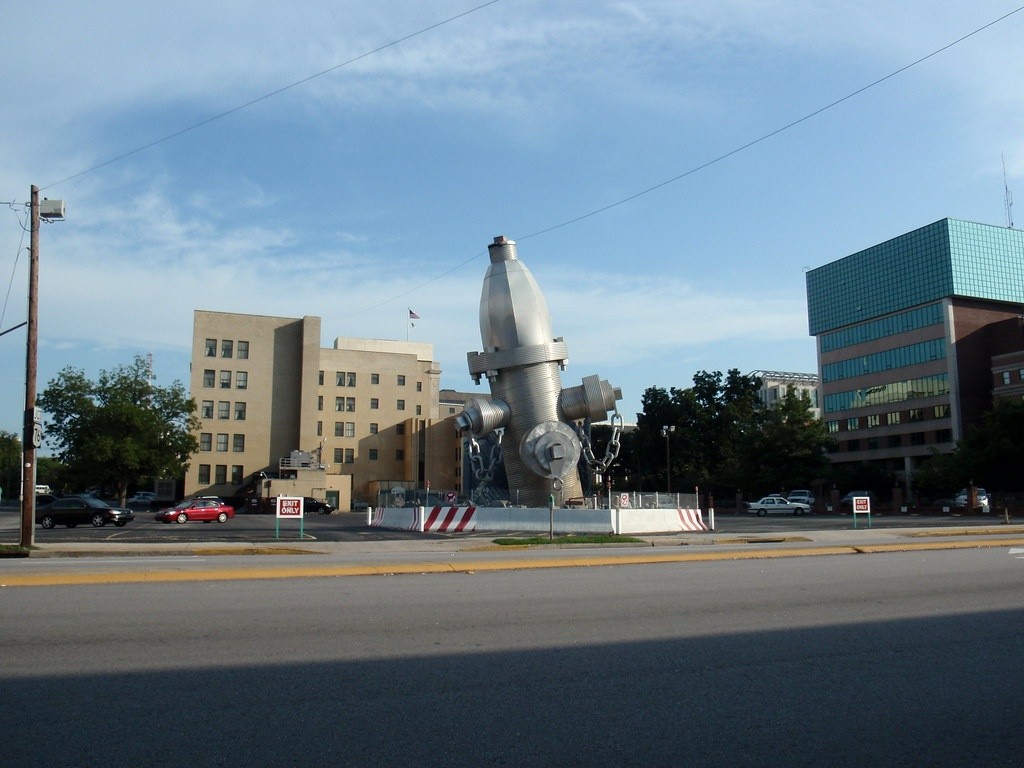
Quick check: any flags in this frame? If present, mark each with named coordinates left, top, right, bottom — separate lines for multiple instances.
left=408, top=308, right=422, bottom=319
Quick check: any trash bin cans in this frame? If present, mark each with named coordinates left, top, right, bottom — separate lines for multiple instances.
left=351, top=500, right=355, bottom=511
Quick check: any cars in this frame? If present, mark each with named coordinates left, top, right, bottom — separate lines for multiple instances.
left=303, top=496, right=335, bottom=516
left=952, top=488, right=992, bottom=513
left=154, top=498, right=235, bottom=524
left=34, top=493, right=136, bottom=529
left=128, top=492, right=156, bottom=506
left=742, top=496, right=813, bottom=518
left=841, top=490, right=872, bottom=504
left=787, top=489, right=816, bottom=505
